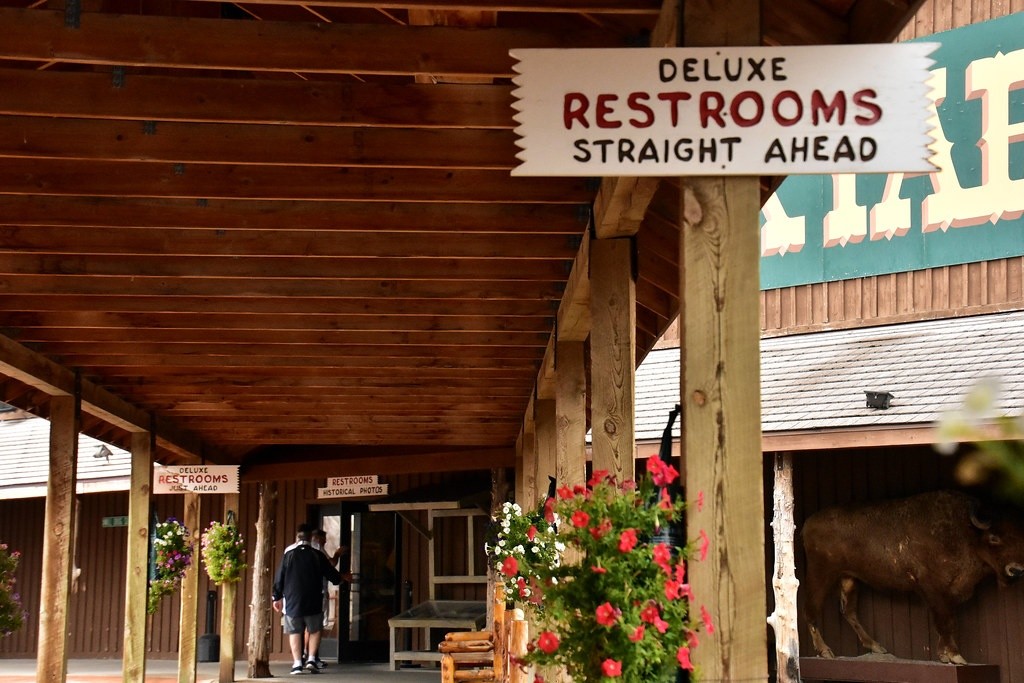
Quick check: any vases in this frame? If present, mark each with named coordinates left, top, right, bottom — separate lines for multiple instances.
left=149, top=533, right=160, bottom=578
left=640, top=528, right=682, bottom=548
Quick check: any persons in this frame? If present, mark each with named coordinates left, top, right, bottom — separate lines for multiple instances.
left=272, top=524, right=352, bottom=675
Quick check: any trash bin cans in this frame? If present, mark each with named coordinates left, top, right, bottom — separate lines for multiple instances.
left=199, top=634, right=219, bottom=662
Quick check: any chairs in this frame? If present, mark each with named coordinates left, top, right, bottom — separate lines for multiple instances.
left=438, top=584, right=529, bottom=682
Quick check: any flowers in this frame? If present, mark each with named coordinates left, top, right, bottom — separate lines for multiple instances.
left=0, top=543, right=29, bottom=638
left=148, top=516, right=195, bottom=615
left=484, top=453, right=714, bottom=683
left=201, top=522, right=249, bottom=585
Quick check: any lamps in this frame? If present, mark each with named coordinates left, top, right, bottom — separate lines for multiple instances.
left=92, top=444, right=113, bottom=459
left=863, top=390, right=894, bottom=409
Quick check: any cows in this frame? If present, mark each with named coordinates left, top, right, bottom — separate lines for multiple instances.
left=802, top=490, right=1024, bottom=666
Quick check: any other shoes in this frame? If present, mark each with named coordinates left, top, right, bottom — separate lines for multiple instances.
left=305, top=661, right=319, bottom=673
left=290, top=666, right=302, bottom=674
left=316, top=662, right=328, bottom=669
